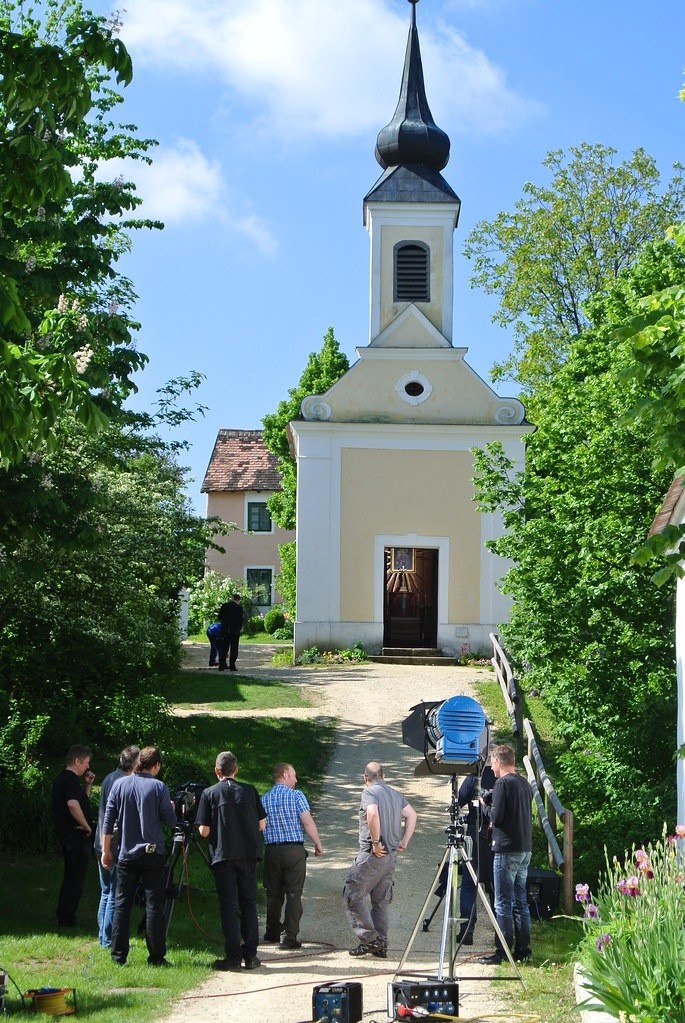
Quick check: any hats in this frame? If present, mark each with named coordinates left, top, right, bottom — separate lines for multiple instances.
left=232, top=593, right=241, bottom=600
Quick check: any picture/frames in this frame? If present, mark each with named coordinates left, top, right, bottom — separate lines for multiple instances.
left=391, top=547, right=417, bottom=574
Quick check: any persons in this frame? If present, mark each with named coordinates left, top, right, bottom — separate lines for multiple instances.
left=52, top=744, right=95, bottom=926
left=260, top=762, right=322, bottom=947
left=101, top=746, right=178, bottom=966
left=196, top=751, right=268, bottom=972
left=479, top=745, right=534, bottom=965
left=208, top=594, right=245, bottom=670
left=457, top=745, right=498, bottom=946
left=341, top=762, right=418, bottom=958
left=93, top=746, right=141, bottom=947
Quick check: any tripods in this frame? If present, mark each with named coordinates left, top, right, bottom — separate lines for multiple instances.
left=163, top=821, right=209, bottom=939
left=393, top=772, right=529, bottom=993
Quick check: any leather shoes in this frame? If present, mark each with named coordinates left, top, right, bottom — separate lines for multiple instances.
left=478, top=949, right=509, bottom=965
left=512, top=950, right=532, bottom=963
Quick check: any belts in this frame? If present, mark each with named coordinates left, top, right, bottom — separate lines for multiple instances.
left=366, top=848, right=371, bottom=853
left=267, top=841, right=303, bottom=845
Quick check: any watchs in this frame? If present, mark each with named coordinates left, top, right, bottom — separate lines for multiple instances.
left=373, top=840, right=380, bottom=846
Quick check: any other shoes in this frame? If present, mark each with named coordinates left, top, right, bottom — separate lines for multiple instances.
left=230, top=668, right=238, bottom=671
left=456, top=929, right=473, bottom=945
left=209, top=662, right=219, bottom=667
left=214, top=958, right=242, bottom=972
left=244, top=957, right=261, bottom=969
left=147, top=955, right=174, bottom=969
left=57, top=920, right=78, bottom=928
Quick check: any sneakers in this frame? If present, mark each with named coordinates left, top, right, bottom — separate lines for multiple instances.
left=279, top=934, right=301, bottom=949
left=264, top=931, right=281, bottom=943
left=349, top=939, right=381, bottom=956
left=371, top=946, right=387, bottom=958
